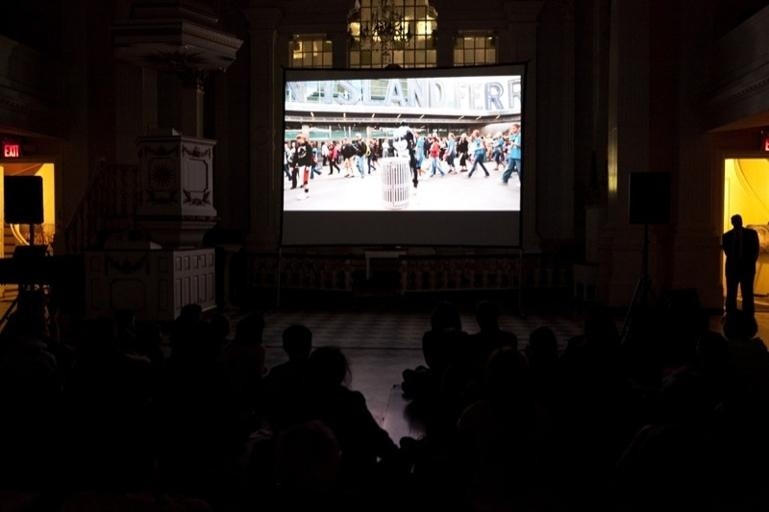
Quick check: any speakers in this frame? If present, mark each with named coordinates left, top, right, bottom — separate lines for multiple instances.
left=4, top=176, right=43, bottom=224
left=628, top=170, right=672, bottom=225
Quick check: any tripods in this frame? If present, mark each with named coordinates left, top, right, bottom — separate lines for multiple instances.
left=0, top=224, right=46, bottom=329
left=618, top=225, right=655, bottom=342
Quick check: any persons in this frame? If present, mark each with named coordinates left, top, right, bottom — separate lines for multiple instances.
left=282, top=124, right=521, bottom=200
left=722, top=214, right=759, bottom=313
